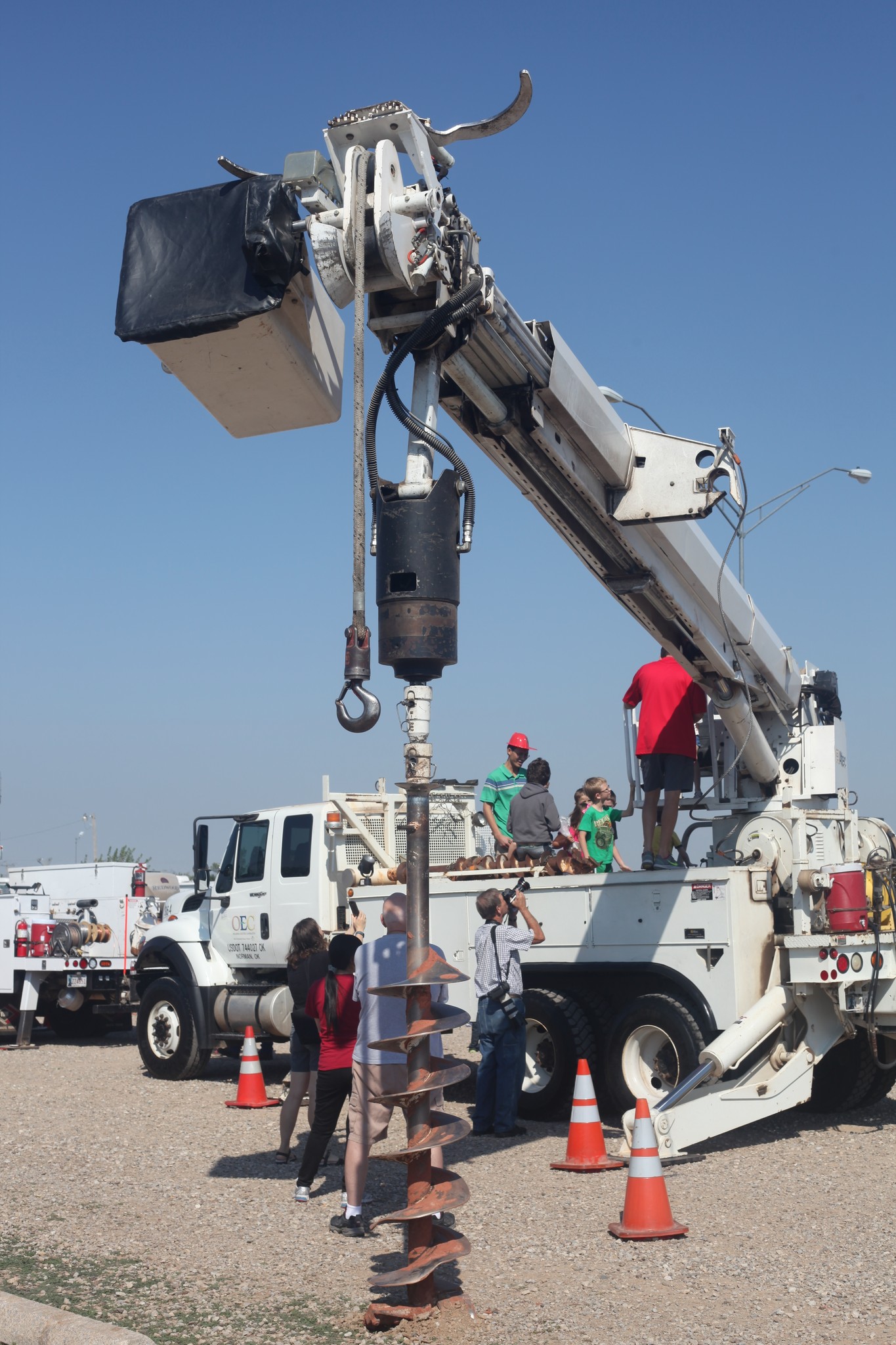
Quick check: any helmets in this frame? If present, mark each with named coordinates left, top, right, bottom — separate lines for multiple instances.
left=506, top=732, right=538, bottom=750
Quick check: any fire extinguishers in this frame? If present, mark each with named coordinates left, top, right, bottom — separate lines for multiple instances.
left=14, top=918, right=29, bottom=957
left=132, top=862, right=148, bottom=897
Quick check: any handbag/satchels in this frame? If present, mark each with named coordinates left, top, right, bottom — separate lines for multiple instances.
left=291, top=1007, right=322, bottom=1045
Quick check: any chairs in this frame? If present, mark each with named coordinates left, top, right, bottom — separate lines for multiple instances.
left=247, top=846, right=265, bottom=877
left=290, top=840, right=309, bottom=875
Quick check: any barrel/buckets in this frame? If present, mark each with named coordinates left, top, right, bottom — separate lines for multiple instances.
left=820, top=864, right=868, bottom=932
left=30, top=919, right=56, bottom=956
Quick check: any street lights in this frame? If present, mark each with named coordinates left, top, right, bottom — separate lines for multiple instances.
left=75, top=831, right=85, bottom=864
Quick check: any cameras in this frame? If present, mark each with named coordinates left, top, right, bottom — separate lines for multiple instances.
left=502, top=876, right=531, bottom=908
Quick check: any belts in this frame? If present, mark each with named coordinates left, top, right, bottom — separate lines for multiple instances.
left=508, top=994, right=520, bottom=998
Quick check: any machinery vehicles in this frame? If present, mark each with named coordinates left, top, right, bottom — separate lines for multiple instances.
left=0, top=65, right=896, bottom=1333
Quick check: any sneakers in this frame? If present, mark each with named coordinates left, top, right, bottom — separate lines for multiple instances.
left=642, top=851, right=654, bottom=870
left=293, top=1178, right=310, bottom=1201
left=653, top=853, right=686, bottom=870
left=329, top=1205, right=365, bottom=1237
left=341, top=1191, right=375, bottom=1208
left=431, top=1211, right=456, bottom=1229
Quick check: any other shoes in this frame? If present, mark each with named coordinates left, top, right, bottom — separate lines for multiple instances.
left=495, top=1125, right=527, bottom=1137
left=474, top=1124, right=495, bottom=1136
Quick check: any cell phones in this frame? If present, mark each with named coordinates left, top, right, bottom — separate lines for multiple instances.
left=349, top=901, right=359, bottom=917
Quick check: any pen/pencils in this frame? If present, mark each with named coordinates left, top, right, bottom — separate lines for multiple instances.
left=510, top=888, right=523, bottom=903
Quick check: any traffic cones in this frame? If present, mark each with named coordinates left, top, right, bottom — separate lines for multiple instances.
left=549, top=1057, right=625, bottom=1172
left=224, top=1026, right=280, bottom=1108
left=607, top=1098, right=691, bottom=1239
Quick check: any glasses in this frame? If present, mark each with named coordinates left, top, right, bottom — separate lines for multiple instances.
left=596, top=785, right=610, bottom=799
left=513, top=750, right=530, bottom=758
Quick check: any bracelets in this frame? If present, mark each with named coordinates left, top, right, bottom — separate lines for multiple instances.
left=356, top=931, right=365, bottom=938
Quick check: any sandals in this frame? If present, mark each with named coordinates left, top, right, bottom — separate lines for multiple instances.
left=318, top=1150, right=345, bottom=1167
left=275, top=1148, right=297, bottom=1164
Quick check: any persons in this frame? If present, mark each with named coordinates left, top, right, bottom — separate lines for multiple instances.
left=474, top=888, right=545, bottom=1138
left=480, top=732, right=537, bottom=854
left=622, top=647, right=708, bottom=870
left=643, top=806, right=698, bottom=869
left=294, top=935, right=364, bottom=1207
left=330, top=893, right=449, bottom=1237
left=276, top=910, right=367, bottom=1167
left=553, top=777, right=635, bottom=875
left=506, top=757, right=561, bottom=877
